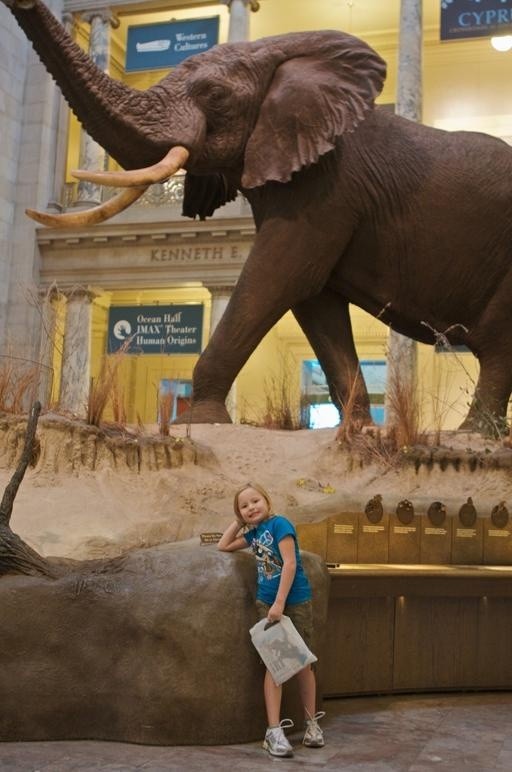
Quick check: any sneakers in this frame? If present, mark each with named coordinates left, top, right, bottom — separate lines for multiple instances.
left=262, top=726, right=294, bottom=758
left=301, top=720, right=324, bottom=748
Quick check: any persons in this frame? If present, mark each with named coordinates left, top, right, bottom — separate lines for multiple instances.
left=218, top=479, right=327, bottom=759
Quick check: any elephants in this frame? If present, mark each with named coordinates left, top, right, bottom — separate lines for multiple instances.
left=0, top=0, right=512, bottom=441
